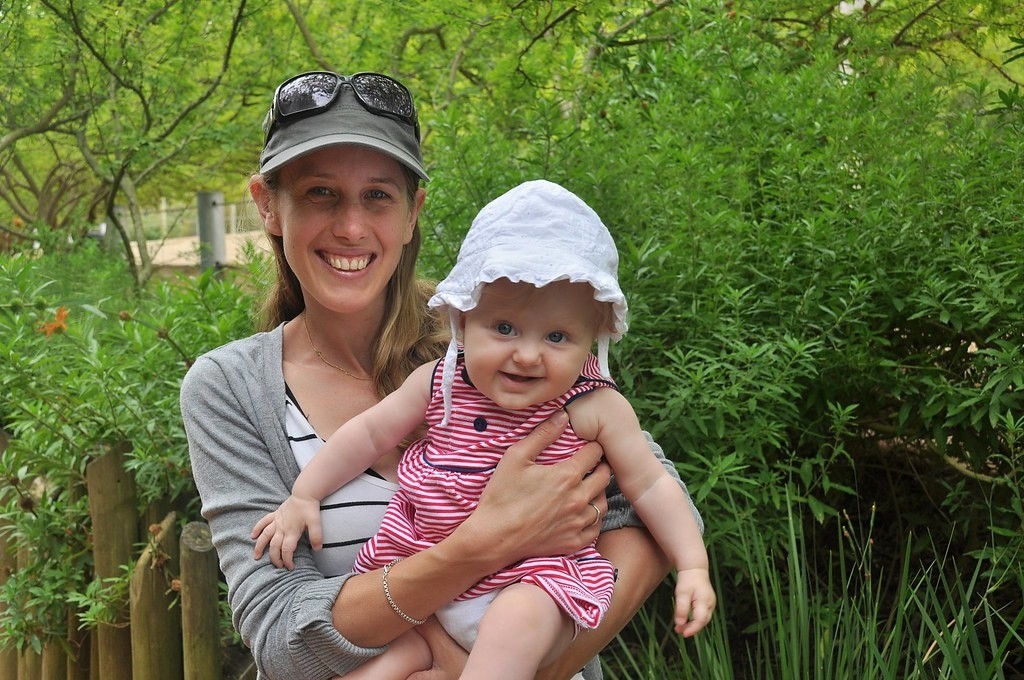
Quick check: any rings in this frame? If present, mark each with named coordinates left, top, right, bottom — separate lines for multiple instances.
left=589, top=503, right=601, bottom=526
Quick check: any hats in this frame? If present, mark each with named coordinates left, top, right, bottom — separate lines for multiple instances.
left=259, top=76, right=431, bottom=182
left=426, top=179, right=629, bottom=427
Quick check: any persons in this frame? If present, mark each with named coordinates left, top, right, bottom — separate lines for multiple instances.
left=251, top=182, right=717, bottom=680
left=180, top=70, right=706, bottom=679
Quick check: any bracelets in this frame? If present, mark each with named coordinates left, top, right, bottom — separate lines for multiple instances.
left=382, top=558, right=427, bottom=625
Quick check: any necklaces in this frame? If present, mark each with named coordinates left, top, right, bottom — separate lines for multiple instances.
left=302, top=310, right=376, bottom=382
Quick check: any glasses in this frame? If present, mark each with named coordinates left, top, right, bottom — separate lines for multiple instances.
left=262, top=71, right=420, bottom=148
